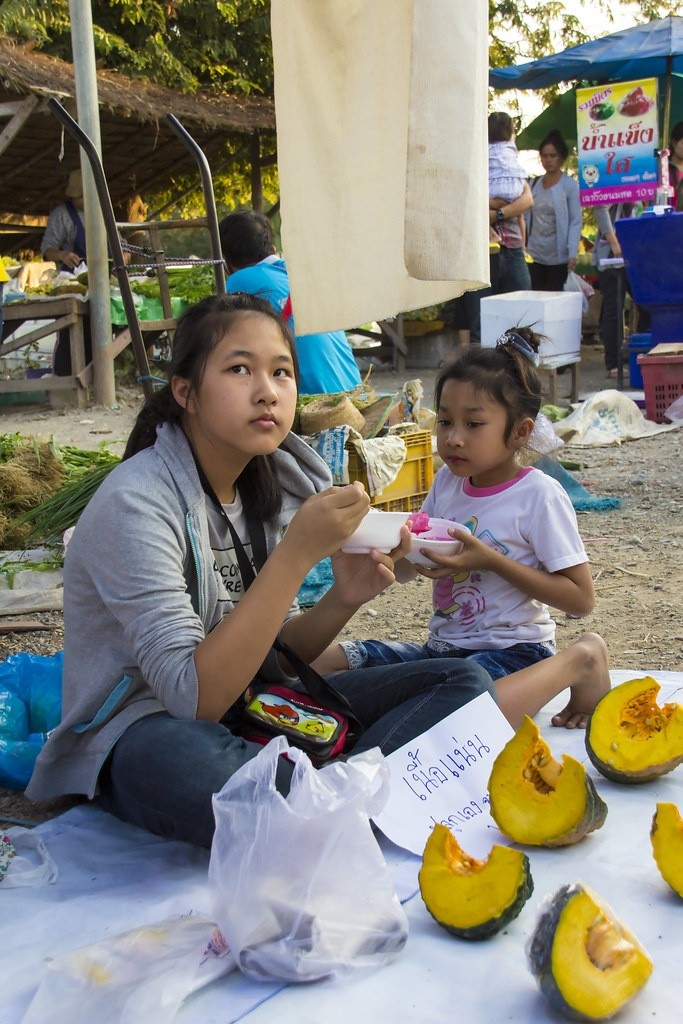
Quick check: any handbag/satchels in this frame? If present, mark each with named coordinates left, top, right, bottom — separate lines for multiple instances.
left=563, top=270, right=595, bottom=316
left=241, top=683, right=349, bottom=764
left=208, top=736, right=409, bottom=981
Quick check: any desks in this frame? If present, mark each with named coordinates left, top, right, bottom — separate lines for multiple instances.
left=1, top=294, right=196, bottom=413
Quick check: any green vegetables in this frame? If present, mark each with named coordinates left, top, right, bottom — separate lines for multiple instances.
left=0, top=431, right=123, bottom=487
left=128, top=264, right=218, bottom=307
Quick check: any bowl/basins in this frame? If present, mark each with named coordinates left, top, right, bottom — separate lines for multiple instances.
left=403, top=517, right=472, bottom=568
left=341, top=510, right=412, bottom=554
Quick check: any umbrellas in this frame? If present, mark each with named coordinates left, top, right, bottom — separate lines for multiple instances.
left=489, top=16, right=683, bottom=149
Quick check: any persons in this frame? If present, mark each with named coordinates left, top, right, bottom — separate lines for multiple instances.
left=487, top=109, right=683, bottom=378
left=220, top=209, right=365, bottom=397
left=22, top=307, right=500, bottom=850
left=304, top=330, right=612, bottom=734
left=40, top=167, right=133, bottom=377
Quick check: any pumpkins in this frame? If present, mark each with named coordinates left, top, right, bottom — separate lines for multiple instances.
left=527, top=882, right=654, bottom=1024
left=584, top=675, right=683, bottom=783
left=417, top=822, right=534, bottom=940
left=487, top=713, right=608, bottom=847
left=650, top=801, right=683, bottom=901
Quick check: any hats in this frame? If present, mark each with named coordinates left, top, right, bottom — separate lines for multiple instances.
left=65, top=168, right=83, bottom=198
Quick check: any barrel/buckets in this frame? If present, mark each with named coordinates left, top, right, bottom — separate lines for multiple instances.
left=26, top=368, right=52, bottom=379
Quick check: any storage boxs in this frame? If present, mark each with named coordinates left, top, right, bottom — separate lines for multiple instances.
left=333, top=429, right=433, bottom=515
left=615, top=208, right=683, bottom=424
left=478, top=287, right=584, bottom=365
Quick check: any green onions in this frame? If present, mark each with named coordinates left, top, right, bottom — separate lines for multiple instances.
left=3, top=458, right=124, bottom=560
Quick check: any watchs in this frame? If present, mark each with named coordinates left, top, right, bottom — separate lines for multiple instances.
left=493, top=207, right=505, bottom=221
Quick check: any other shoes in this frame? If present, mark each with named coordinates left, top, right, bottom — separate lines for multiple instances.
left=609, top=366, right=631, bottom=378
left=490, top=242, right=501, bottom=254
left=525, top=253, right=534, bottom=263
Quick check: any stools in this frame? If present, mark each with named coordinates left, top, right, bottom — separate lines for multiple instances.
left=532, top=362, right=577, bottom=407
